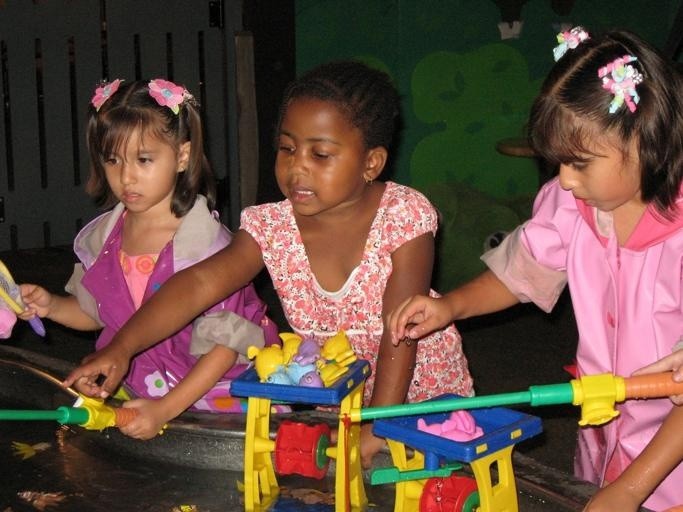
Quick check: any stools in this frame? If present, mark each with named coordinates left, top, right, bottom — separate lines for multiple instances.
left=497, top=137, right=559, bottom=192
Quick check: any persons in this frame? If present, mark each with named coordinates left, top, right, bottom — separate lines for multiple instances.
left=631, top=346, right=683, bottom=407
left=15, top=78, right=293, bottom=442
left=62, top=58, right=477, bottom=471
left=385, top=27, right=683, bottom=511
